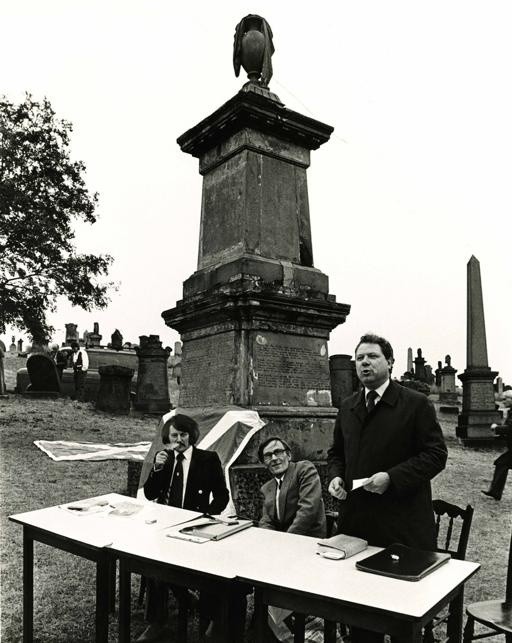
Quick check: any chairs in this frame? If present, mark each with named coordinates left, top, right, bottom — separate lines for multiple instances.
left=463, top=536, right=512, bottom=643
left=138, top=575, right=209, bottom=642
left=261, top=508, right=339, bottom=642
left=432, top=499, right=474, bottom=643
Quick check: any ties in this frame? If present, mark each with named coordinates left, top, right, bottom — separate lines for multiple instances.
left=367, top=390, right=379, bottom=415
left=168, top=454, right=185, bottom=508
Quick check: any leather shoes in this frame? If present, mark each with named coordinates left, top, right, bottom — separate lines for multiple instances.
left=135, top=623, right=168, bottom=643
left=481, top=490, right=501, bottom=501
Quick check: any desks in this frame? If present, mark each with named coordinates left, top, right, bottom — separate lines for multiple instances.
left=234, top=531, right=482, bottom=643
left=8, top=493, right=204, bottom=642
left=103, top=512, right=327, bottom=642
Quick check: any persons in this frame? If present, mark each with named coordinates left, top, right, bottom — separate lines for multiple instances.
left=480, top=390, right=512, bottom=500
left=328, top=334, right=447, bottom=643
left=49, top=344, right=68, bottom=383
left=136, top=414, right=229, bottom=642
left=204, top=435, right=328, bottom=638
left=68, top=342, right=89, bottom=402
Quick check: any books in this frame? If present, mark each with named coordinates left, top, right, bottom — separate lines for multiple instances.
left=163, top=513, right=252, bottom=544
left=316, top=533, right=368, bottom=559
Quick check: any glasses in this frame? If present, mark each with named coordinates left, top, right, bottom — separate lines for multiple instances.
left=262, top=449, right=286, bottom=461
